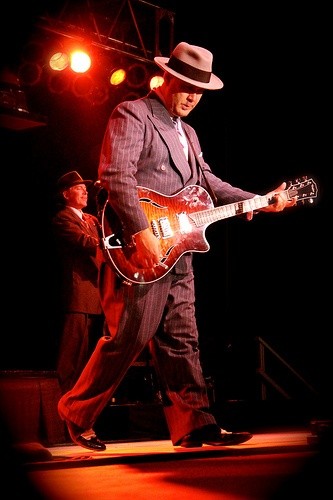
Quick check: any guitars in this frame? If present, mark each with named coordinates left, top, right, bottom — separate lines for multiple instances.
left=99, top=174, right=319, bottom=286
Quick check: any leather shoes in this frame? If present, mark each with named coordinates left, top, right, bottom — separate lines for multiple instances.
left=58, top=406, right=107, bottom=452
left=180, top=424, right=253, bottom=448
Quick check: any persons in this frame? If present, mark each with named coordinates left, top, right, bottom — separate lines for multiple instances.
left=56, top=40, right=297, bottom=452
left=53, top=170, right=116, bottom=444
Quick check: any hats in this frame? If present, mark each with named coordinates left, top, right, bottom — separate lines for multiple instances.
left=56, top=171, right=93, bottom=190
left=153, top=42, right=224, bottom=91
